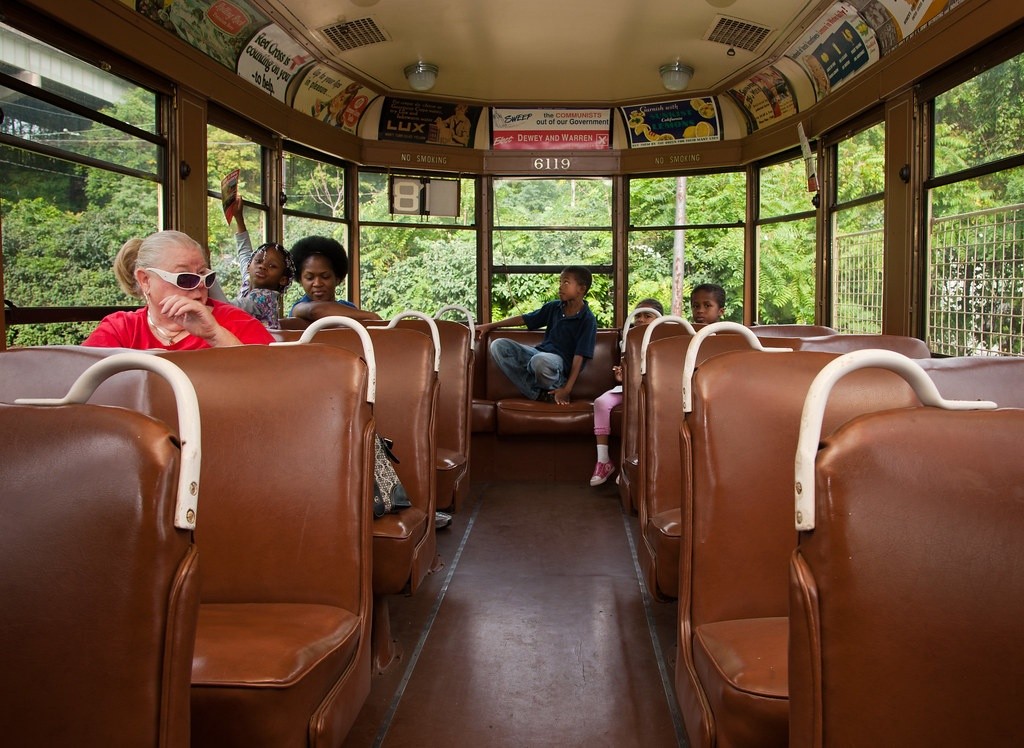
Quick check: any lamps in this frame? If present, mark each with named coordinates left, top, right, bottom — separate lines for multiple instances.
left=660, top=64, right=696, bottom=92
left=403, top=62, right=438, bottom=93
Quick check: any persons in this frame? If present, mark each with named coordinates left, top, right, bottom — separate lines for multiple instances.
left=472, top=265, right=597, bottom=405
left=590, top=299, right=664, bottom=485
left=689, top=283, right=726, bottom=325
left=285, top=235, right=382, bottom=322
left=77, top=230, right=277, bottom=351
left=436, top=102, right=470, bottom=148
left=803, top=55, right=831, bottom=102
left=866, top=3, right=897, bottom=50
left=229, top=195, right=296, bottom=330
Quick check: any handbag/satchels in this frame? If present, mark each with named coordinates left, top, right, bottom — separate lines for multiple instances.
left=371, top=434, right=411, bottom=514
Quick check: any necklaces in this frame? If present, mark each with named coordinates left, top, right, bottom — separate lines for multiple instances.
left=147, top=308, right=184, bottom=346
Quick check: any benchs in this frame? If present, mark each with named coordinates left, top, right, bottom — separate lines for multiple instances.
left=788, top=349, right=1024, bottom=748
left=620, top=308, right=1024, bottom=748
left=638, top=314, right=1024, bottom=748
left=459, top=322, right=623, bottom=481
left=679, top=321, right=1024, bottom=748
left=0, top=351, right=202, bottom=748
left=0, top=310, right=442, bottom=748
left=0, top=305, right=475, bottom=748
left=0, top=315, right=376, bottom=748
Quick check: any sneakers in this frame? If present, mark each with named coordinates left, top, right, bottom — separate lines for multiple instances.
left=436, top=512, right=452, bottom=528
left=590, top=460, right=615, bottom=485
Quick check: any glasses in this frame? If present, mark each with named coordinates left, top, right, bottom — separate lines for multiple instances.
left=146, top=268, right=216, bottom=290
